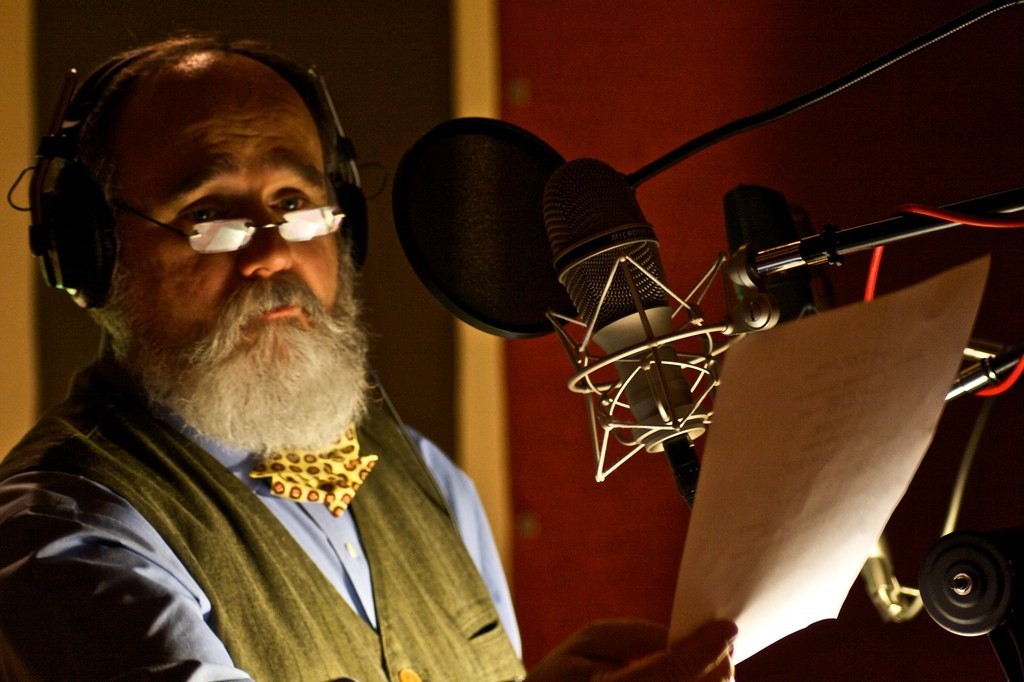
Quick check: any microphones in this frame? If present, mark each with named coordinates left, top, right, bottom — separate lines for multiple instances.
left=722, top=185, right=909, bottom=627
left=542, top=157, right=706, bottom=510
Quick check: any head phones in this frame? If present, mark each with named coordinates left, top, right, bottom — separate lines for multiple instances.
left=27, top=39, right=368, bottom=309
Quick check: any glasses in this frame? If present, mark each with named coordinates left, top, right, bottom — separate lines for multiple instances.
left=112, top=174, right=349, bottom=254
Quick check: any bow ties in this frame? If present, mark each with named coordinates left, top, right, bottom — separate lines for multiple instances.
left=247, top=421, right=379, bottom=517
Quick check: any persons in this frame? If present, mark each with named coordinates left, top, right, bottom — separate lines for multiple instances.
left=0, top=35, right=738, bottom=682
